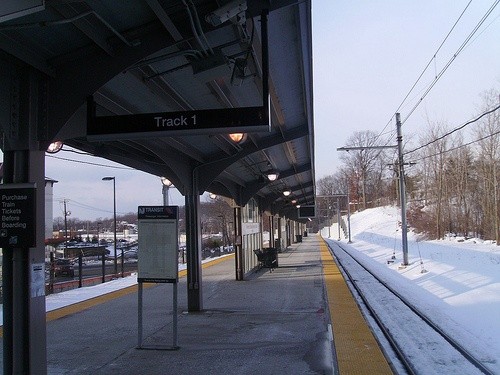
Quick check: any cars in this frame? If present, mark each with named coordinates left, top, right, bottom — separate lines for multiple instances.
left=54, top=259, right=75, bottom=278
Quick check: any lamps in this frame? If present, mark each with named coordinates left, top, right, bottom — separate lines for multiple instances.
left=283, top=188, right=292, bottom=197
left=229, top=132, right=247, bottom=144
left=291, top=198, right=301, bottom=209
left=266, top=170, right=278, bottom=182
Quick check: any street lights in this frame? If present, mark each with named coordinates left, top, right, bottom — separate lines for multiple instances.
left=97, top=223, right=103, bottom=260
left=101, top=177, right=117, bottom=274
